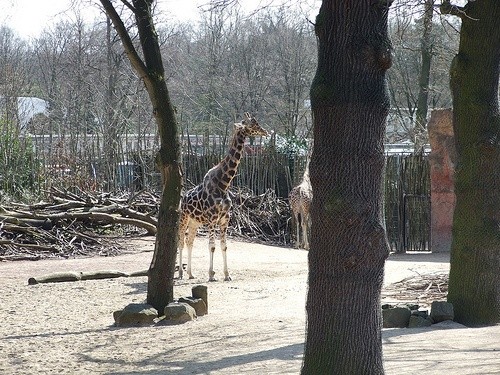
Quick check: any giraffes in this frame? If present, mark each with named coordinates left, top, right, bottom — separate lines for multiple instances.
left=178, top=113, right=269, bottom=281
left=290, top=138, right=313, bottom=251
